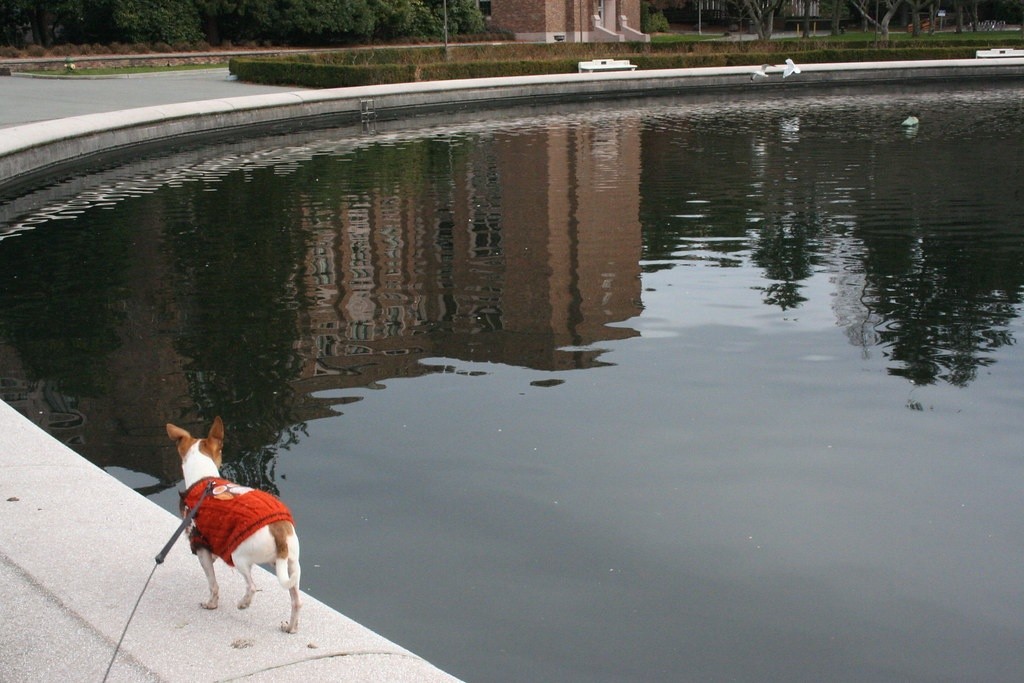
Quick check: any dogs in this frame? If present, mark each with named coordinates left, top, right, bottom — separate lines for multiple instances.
left=165, top=414, right=303, bottom=634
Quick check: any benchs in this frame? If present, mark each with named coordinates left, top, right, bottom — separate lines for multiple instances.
left=578, top=59, right=638, bottom=74
left=976, top=48, right=1024, bottom=59
